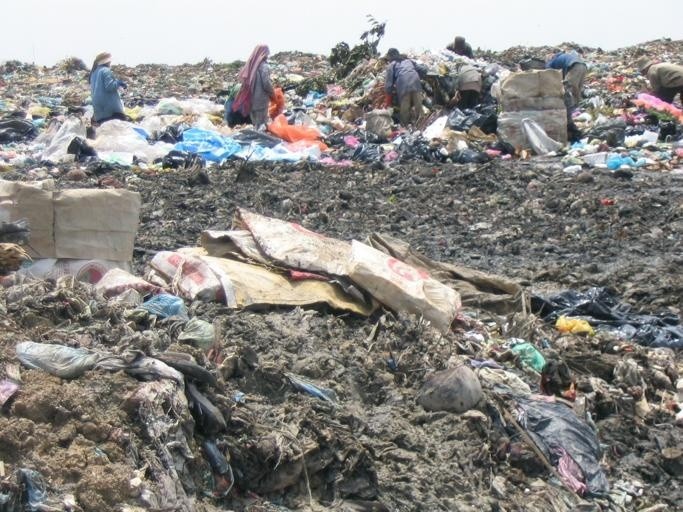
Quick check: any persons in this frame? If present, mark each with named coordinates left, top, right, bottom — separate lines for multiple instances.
left=89, top=52, right=128, bottom=125
left=447, top=36, right=473, bottom=58
left=231, top=44, right=274, bottom=131
left=636, top=54, right=683, bottom=107
left=224, top=83, right=252, bottom=129
left=384, top=48, right=428, bottom=126
left=545, top=49, right=587, bottom=104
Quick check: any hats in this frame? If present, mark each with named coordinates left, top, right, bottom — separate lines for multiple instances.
left=383, top=48, right=407, bottom=61
left=637, top=55, right=654, bottom=71
